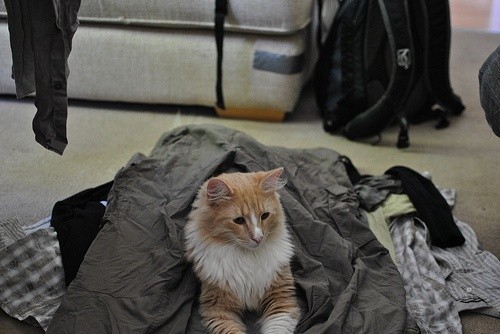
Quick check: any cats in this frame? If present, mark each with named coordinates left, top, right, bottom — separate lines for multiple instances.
left=183, top=166, right=301, bottom=334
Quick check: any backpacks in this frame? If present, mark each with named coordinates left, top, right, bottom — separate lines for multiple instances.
left=308, top=0, right=466, bottom=139
